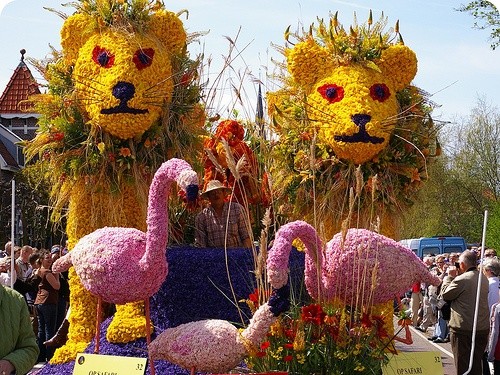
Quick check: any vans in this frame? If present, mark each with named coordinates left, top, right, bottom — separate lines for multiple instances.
left=398, top=236, right=467, bottom=262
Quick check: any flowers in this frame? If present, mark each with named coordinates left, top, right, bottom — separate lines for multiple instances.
left=209, top=132, right=414, bottom=374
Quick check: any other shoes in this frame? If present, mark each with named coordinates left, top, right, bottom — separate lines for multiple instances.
left=414, top=326, right=423, bottom=331
left=433, top=339, right=444, bottom=343
left=429, top=337, right=437, bottom=341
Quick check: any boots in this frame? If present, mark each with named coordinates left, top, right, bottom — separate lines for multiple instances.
left=43, top=319, right=69, bottom=346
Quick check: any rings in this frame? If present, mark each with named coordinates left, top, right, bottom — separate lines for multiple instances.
left=3, top=371, right=5, bottom=375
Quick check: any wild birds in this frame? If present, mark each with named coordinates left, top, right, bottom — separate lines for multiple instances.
left=267, top=220, right=442, bottom=350
left=146, top=296, right=282, bottom=374
left=50, top=157, right=202, bottom=374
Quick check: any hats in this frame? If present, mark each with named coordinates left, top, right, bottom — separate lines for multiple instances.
left=201, top=180, right=232, bottom=197
left=51, top=247, right=61, bottom=253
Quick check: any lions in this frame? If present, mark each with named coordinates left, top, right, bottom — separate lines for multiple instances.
left=21, top=1, right=224, bottom=365
left=260, top=10, right=441, bottom=347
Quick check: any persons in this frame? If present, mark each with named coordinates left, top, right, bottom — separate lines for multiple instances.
left=195, top=180, right=253, bottom=248
left=0, top=241, right=72, bottom=365
left=393, top=247, right=500, bottom=375
left=0, top=283, right=40, bottom=375
